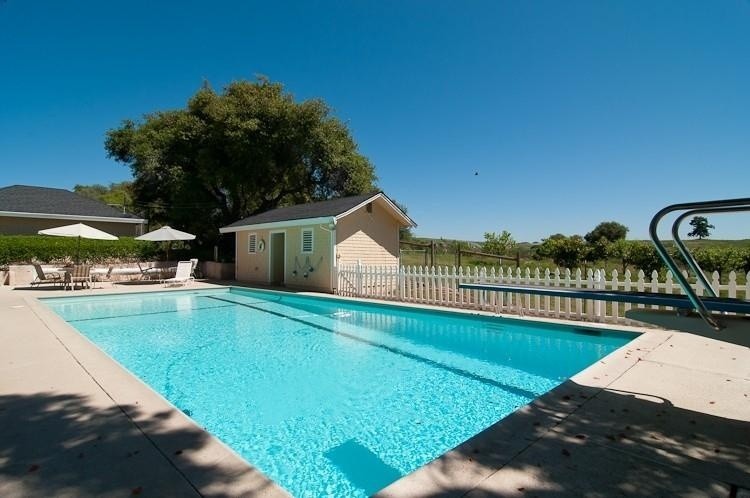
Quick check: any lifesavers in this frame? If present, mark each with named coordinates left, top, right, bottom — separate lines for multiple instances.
left=257, top=240, right=265, bottom=253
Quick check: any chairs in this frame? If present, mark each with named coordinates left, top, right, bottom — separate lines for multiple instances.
left=136, top=257, right=200, bottom=288
left=30, top=260, right=115, bottom=291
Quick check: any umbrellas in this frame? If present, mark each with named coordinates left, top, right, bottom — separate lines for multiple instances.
left=37, top=222, right=119, bottom=266
left=134, top=225, right=196, bottom=261
